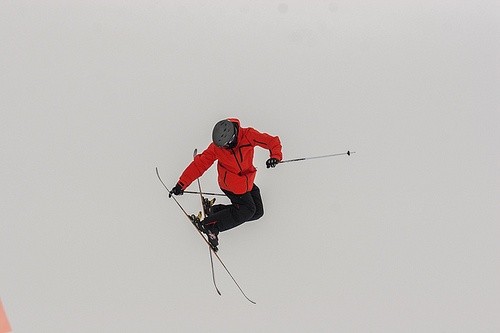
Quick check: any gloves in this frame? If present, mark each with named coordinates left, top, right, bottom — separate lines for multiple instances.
left=266, top=158, right=279, bottom=168
left=169, top=183, right=184, bottom=198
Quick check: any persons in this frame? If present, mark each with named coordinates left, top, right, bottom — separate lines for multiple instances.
left=168, top=118, right=283, bottom=248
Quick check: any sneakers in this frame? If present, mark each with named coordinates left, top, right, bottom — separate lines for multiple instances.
left=198, top=217, right=218, bottom=247
left=204, top=206, right=214, bottom=216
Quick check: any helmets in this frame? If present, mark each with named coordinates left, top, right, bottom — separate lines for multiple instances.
left=212, top=120, right=234, bottom=147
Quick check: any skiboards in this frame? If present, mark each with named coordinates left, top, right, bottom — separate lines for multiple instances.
left=155, top=149, right=256, bottom=304
